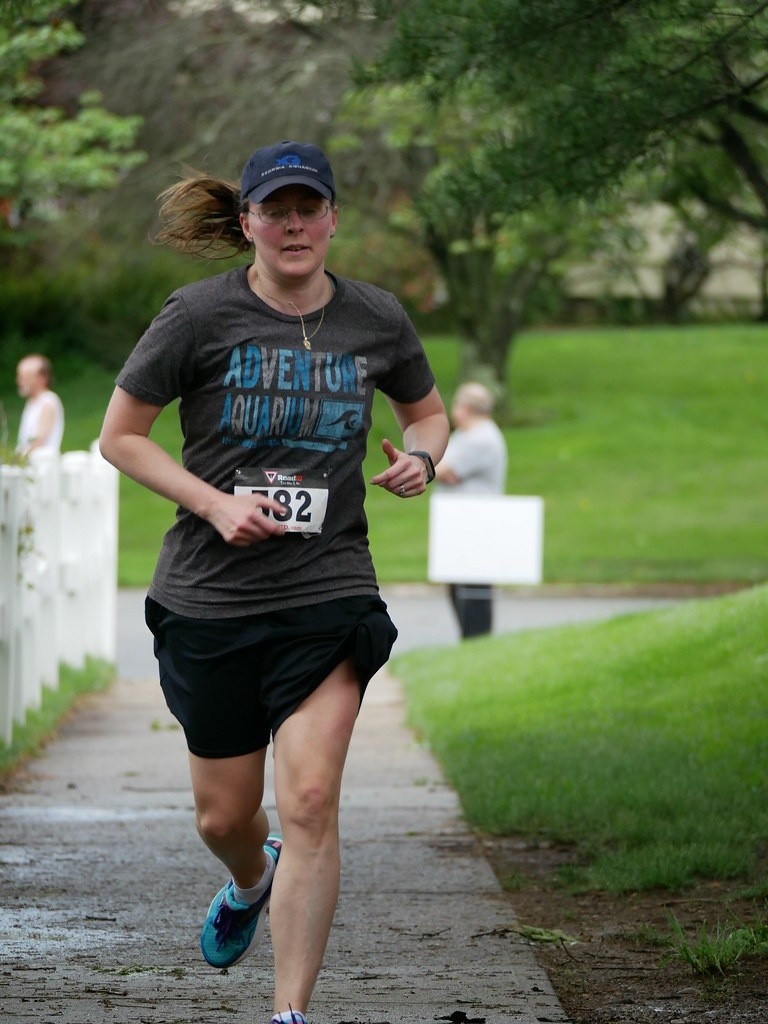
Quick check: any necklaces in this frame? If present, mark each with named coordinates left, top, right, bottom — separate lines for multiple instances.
left=257, top=277, right=326, bottom=349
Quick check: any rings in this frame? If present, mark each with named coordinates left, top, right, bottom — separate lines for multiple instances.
left=401, top=483, right=406, bottom=496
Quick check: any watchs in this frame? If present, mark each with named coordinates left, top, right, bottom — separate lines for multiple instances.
left=409, top=451, right=435, bottom=484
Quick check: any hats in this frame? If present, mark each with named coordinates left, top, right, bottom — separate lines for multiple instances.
left=240, top=141, right=336, bottom=206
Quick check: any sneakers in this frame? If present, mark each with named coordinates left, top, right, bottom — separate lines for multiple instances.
left=201, top=831, right=283, bottom=968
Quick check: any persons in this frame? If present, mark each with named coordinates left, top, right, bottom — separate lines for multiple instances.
left=430, top=381, right=507, bottom=641
left=97, top=139, right=451, bottom=1024
left=14, top=351, right=65, bottom=462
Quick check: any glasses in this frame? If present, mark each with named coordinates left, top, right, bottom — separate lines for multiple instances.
left=248, top=201, right=332, bottom=224
